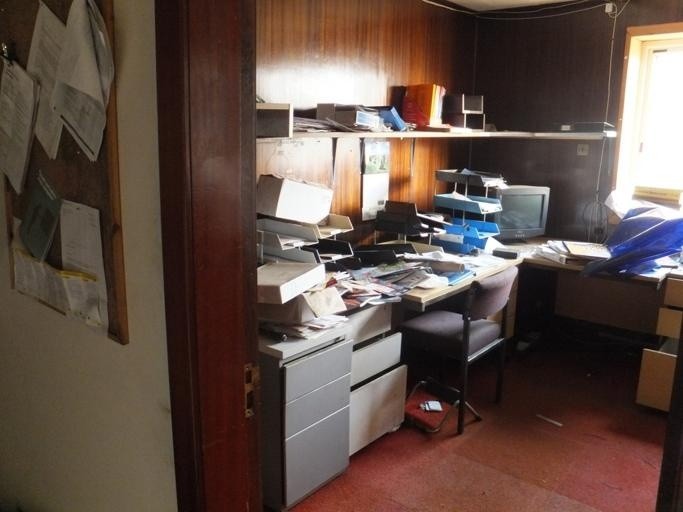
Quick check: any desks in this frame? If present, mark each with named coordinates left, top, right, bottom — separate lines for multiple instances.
left=527, top=228, right=683, bottom=409
left=328, top=229, right=523, bottom=457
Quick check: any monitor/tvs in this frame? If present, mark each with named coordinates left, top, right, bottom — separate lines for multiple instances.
left=494, top=185, right=550, bottom=245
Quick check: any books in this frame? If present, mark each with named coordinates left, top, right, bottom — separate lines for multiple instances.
left=535, top=239, right=612, bottom=268
left=403, top=82, right=445, bottom=125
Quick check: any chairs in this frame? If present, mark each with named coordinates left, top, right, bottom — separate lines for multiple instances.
left=397, top=259, right=521, bottom=437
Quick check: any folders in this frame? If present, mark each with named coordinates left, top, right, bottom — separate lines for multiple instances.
left=368, top=106, right=407, bottom=132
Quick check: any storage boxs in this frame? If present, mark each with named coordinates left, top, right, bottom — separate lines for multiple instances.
left=254, top=172, right=335, bottom=223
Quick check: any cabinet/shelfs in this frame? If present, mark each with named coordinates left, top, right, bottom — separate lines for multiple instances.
left=240, top=86, right=618, bottom=164
left=261, top=332, right=350, bottom=507
